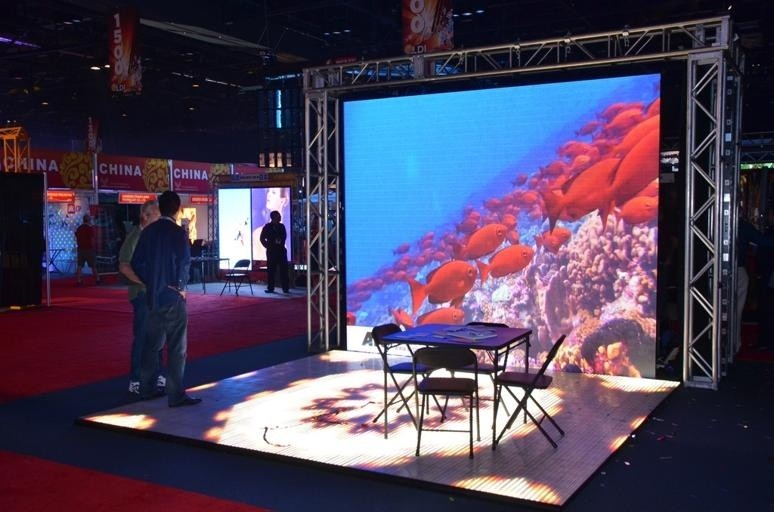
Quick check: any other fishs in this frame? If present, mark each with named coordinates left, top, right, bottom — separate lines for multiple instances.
left=346, top=79, right=661, bottom=317
left=414, top=306, right=470, bottom=329
left=346, top=79, right=661, bottom=329
left=385, top=304, right=413, bottom=331
left=346, top=313, right=356, bottom=323
left=405, top=259, right=478, bottom=313
left=477, top=244, right=536, bottom=285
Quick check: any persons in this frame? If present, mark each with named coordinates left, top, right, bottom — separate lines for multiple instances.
left=253, top=187, right=289, bottom=261
left=116, top=199, right=168, bottom=398
left=733, top=169, right=774, bottom=358
left=74, top=213, right=104, bottom=287
left=258, top=210, right=290, bottom=294
left=131, top=192, right=201, bottom=407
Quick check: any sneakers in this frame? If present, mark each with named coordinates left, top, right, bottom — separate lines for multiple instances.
left=127, top=374, right=168, bottom=400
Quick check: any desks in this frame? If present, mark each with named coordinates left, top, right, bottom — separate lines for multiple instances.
left=190, top=257, right=229, bottom=294
left=382, top=323, right=532, bottom=450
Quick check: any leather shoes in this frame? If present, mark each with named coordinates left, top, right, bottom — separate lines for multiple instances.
left=167, top=395, right=203, bottom=409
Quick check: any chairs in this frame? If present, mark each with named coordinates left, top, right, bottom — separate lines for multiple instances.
left=493, top=334, right=566, bottom=447
left=220, top=260, right=253, bottom=297
left=441, top=322, right=510, bottom=423
left=371, top=323, right=428, bottom=430
left=412, top=346, right=480, bottom=458
